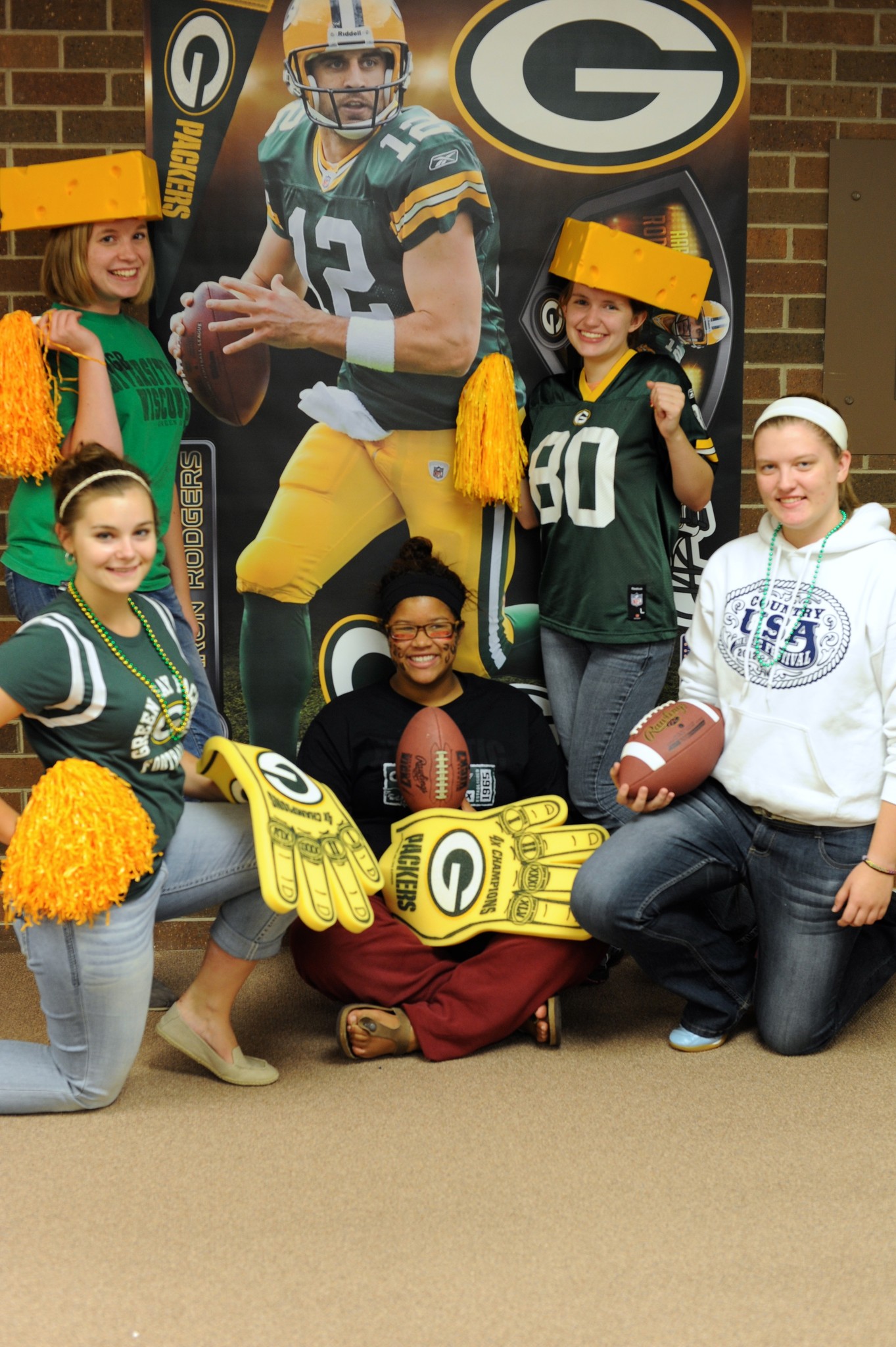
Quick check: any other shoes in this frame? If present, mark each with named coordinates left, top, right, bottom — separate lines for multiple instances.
left=156, top=1002, right=278, bottom=1085
left=668, top=1024, right=728, bottom=1052
left=148, top=980, right=178, bottom=1011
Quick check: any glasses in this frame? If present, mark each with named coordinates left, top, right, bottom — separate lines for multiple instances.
left=389, top=622, right=453, bottom=640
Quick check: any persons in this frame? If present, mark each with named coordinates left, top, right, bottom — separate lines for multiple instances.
left=570, top=395, right=896, bottom=1056
left=280, top=535, right=579, bottom=1060
left=628, top=301, right=730, bottom=363
left=455, top=277, right=720, bottom=833
left=1, top=444, right=297, bottom=1115
left=0, top=218, right=223, bottom=755
left=168, top=0, right=528, bottom=758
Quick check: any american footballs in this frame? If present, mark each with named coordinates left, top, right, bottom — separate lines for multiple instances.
left=618, top=697, right=724, bottom=796
left=395, top=706, right=470, bottom=809
left=179, top=280, right=271, bottom=426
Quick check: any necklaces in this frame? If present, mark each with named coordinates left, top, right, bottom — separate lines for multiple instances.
left=68, top=578, right=190, bottom=742
left=755, top=510, right=847, bottom=666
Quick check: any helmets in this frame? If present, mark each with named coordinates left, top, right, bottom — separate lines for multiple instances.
left=670, top=300, right=730, bottom=349
left=282, top=0, right=412, bottom=113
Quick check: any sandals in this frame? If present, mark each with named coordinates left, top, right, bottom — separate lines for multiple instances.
left=335, top=1003, right=410, bottom=1060
left=530, top=997, right=562, bottom=1047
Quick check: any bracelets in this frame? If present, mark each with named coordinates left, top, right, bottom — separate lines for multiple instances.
left=862, top=855, right=896, bottom=875
left=344, top=316, right=394, bottom=373
